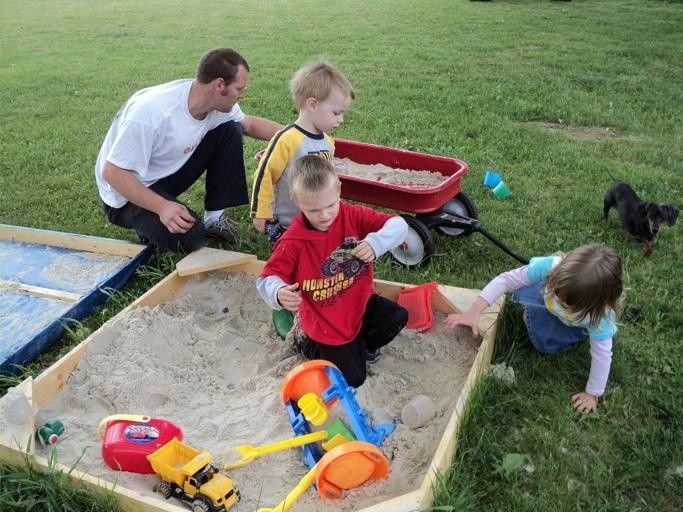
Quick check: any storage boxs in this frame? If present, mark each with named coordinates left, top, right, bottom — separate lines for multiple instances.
left=98, top=414, right=183, bottom=474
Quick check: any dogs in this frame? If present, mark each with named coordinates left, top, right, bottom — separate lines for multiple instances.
left=602, top=166, right=679, bottom=259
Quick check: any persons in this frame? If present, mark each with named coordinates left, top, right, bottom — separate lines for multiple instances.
left=248, top=62, right=355, bottom=245
left=255, top=154, right=409, bottom=391
left=94, top=46, right=284, bottom=257
left=443, top=243, right=624, bottom=418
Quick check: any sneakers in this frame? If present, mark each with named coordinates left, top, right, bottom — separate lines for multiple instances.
left=203, top=211, right=256, bottom=250
left=365, top=348, right=380, bottom=364
left=285, top=312, right=304, bottom=355
